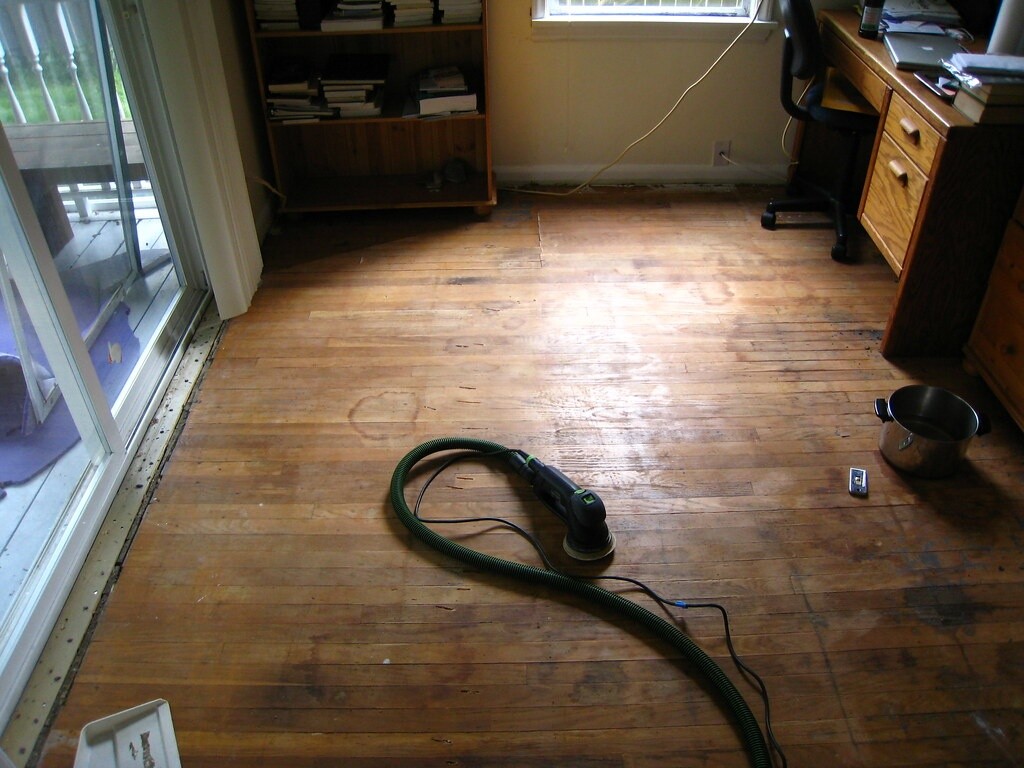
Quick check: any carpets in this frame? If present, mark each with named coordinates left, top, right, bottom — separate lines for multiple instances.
left=0, top=276, right=140, bottom=496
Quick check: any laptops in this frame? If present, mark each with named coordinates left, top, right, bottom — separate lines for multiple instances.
left=882, top=30, right=967, bottom=72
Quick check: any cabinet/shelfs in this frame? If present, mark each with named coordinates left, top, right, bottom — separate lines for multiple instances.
left=246, top=0, right=496, bottom=216
left=784, top=10, right=1023, bottom=434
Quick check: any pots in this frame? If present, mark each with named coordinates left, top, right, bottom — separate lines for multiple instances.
left=874, top=384, right=980, bottom=479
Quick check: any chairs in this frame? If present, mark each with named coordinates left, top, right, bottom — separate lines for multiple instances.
left=762, top=1, right=880, bottom=264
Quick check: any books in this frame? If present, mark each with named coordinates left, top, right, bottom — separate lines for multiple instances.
left=266, top=77, right=333, bottom=125
left=400, top=68, right=479, bottom=119
left=439, top=0, right=482, bottom=23
left=860, top=0, right=961, bottom=36
left=319, top=77, right=385, bottom=118
left=942, top=51, right=1024, bottom=124
left=385, top=0, right=434, bottom=27
left=320, top=0, right=382, bottom=31
left=254, top=0, right=300, bottom=31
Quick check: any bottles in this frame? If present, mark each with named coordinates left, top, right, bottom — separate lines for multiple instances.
left=857, top=0, right=885, bottom=40
